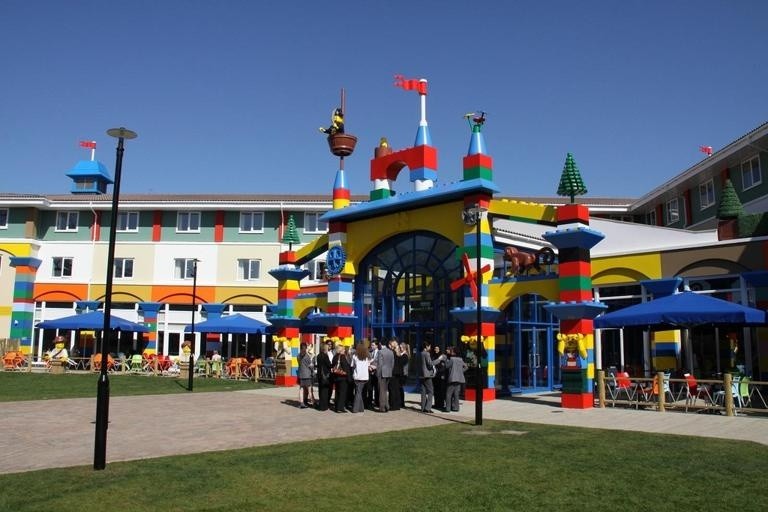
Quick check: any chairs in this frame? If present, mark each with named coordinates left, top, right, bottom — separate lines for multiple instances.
left=602, top=364, right=753, bottom=416
left=0, top=350, right=276, bottom=379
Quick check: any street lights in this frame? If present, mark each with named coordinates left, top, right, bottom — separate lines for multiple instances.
left=464, top=204, right=489, bottom=423
left=185, top=256, right=205, bottom=393
left=91, top=123, right=138, bottom=469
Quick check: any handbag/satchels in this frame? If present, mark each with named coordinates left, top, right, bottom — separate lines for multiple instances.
left=331, top=366, right=347, bottom=375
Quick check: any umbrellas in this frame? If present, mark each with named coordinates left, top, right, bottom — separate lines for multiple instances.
left=591, top=290, right=766, bottom=378
left=184, top=313, right=276, bottom=359
left=34, top=309, right=149, bottom=355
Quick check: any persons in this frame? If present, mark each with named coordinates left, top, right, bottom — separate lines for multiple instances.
left=212, top=350, right=224, bottom=361
left=297, top=335, right=469, bottom=412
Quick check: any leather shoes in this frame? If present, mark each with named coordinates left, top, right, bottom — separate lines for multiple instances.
left=337, top=410, right=347, bottom=413
